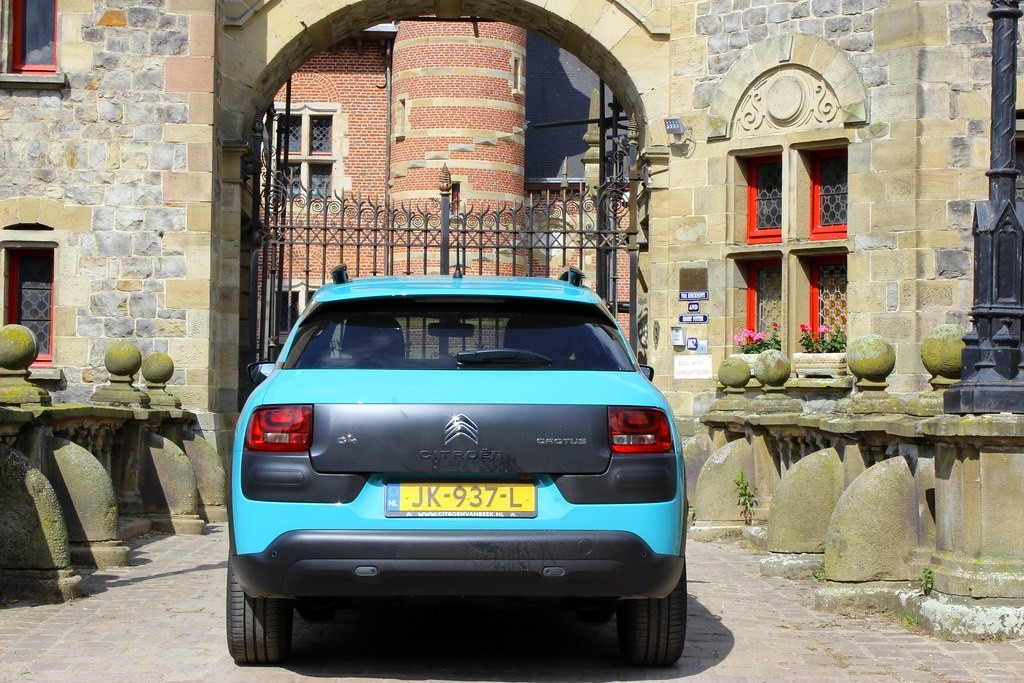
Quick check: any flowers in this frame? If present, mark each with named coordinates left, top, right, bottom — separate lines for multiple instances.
left=734, top=319, right=782, bottom=354
left=798, top=315, right=848, bottom=352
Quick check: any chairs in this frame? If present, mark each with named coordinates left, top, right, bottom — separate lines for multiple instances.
left=503, top=310, right=577, bottom=362
left=341, top=314, right=407, bottom=369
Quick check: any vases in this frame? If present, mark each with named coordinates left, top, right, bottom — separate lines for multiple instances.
left=793, top=351, right=848, bottom=379
left=729, top=352, right=762, bottom=378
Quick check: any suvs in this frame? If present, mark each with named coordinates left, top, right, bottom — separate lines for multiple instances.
left=225, top=262, right=690, bottom=666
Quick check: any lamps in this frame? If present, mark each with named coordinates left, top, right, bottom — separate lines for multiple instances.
left=663, top=116, right=692, bottom=134
